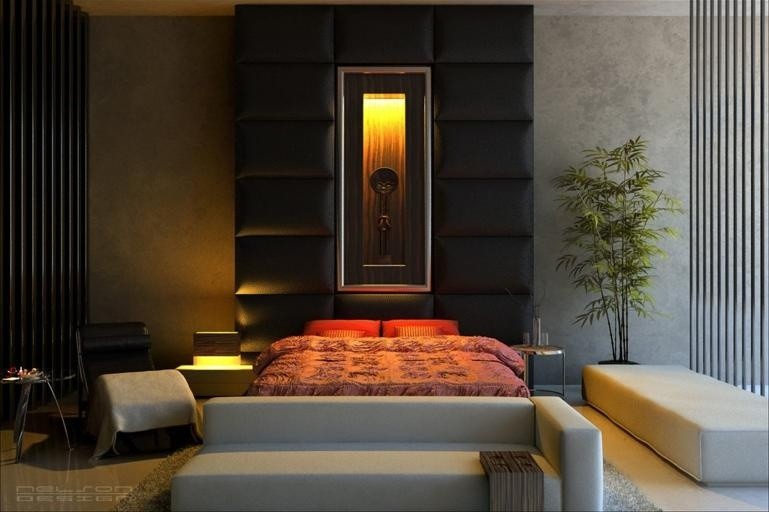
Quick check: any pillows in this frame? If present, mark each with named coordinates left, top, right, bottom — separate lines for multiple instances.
left=381, top=319, right=461, bottom=337
left=302, top=318, right=381, bottom=338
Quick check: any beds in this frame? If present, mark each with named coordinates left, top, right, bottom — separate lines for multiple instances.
left=247, top=335, right=531, bottom=398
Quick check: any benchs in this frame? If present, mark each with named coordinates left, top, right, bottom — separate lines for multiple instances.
left=583, top=362, right=768, bottom=487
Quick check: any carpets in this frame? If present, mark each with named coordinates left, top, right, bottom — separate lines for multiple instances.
left=107, top=442, right=662, bottom=512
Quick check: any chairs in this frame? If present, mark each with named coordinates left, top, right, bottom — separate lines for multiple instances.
left=75, top=319, right=203, bottom=462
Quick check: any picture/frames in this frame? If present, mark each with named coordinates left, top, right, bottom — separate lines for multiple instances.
left=337, top=65, right=433, bottom=293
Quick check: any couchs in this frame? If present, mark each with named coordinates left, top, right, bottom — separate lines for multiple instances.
left=168, top=395, right=603, bottom=511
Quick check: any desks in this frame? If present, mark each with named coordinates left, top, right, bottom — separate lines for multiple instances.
left=0, top=365, right=80, bottom=464
left=510, top=343, right=568, bottom=399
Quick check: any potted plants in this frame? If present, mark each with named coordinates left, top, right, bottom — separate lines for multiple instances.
left=546, top=133, right=687, bottom=403
left=502, top=281, right=547, bottom=347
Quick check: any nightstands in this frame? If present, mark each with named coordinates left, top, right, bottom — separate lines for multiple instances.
left=174, top=364, right=256, bottom=397
